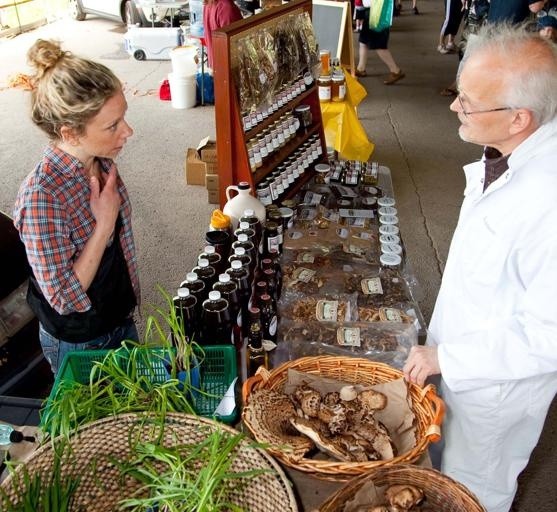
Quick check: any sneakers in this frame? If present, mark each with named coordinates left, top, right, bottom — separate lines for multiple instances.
left=348, top=68, right=367, bottom=77
left=437, top=43, right=450, bottom=54
left=383, top=69, right=405, bottom=84
left=446, top=40, right=456, bottom=50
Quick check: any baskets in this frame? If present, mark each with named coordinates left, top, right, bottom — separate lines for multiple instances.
left=39, top=344, right=239, bottom=440
left=242, top=354, right=446, bottom=485
left=311, top=462, right=487, bottom=511
left=0, top=411, right=301, bottom=511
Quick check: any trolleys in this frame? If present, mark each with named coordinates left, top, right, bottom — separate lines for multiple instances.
left=123, top=26, right=183, bottom=63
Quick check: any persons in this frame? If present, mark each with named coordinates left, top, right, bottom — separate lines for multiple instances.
left=402, top=27, right=556, bottom=511
left=12, top=37, right=141, bottom=387
left=350, top=1, right=404, bottom=86
left=203, top=0, right=245, bottom=69
left=436, top=0, right=557, bottom=98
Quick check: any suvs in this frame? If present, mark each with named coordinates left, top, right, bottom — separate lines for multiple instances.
left=67, top=0, right=190, bottom=27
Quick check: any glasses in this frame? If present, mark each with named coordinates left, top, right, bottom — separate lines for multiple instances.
left=454, top=79, right=520, bottom=121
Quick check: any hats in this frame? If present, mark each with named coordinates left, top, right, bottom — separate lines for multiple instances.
left=536, top=15, right=556, bottom=26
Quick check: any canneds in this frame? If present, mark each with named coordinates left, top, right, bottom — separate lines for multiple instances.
left=318, top=74, right=347, bottom=103
left=294, top=104, right=313, bottom=128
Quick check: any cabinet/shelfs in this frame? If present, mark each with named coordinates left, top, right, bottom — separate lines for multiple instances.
left=210, top=0, right=330, bottom=215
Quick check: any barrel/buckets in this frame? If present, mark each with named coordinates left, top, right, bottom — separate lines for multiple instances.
left=169, top=46, right=197, bottom=77
left=222, top=180, right=267, bottom=253
left=168, top=73, right=197, bottom=110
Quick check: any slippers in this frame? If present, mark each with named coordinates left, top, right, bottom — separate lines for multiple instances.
left=441, top=88, right=456, bottom=97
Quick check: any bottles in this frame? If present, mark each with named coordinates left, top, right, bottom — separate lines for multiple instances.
left=0, top=422, right=24, bottom=446
left=166, top=203, right=284, bottom=379
left=222, top=181, right=266, bottom=231
left=316, top=50, right=346, bottom=104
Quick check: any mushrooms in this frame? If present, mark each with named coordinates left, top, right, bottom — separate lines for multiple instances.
left=241, top=381, right=426, bottom=512
